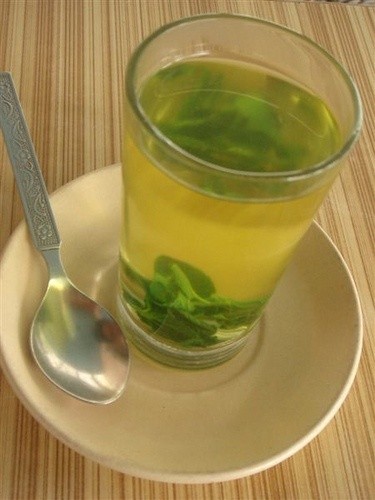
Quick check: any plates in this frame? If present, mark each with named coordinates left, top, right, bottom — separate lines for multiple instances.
left=1, top=157, right=363, bottom=486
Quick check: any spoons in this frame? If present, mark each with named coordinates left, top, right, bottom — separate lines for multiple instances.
left=1, top=70, right=131, bottom=405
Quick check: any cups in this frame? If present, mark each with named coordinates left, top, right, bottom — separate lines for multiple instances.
left=111, top=10, right=362, bottom=369
